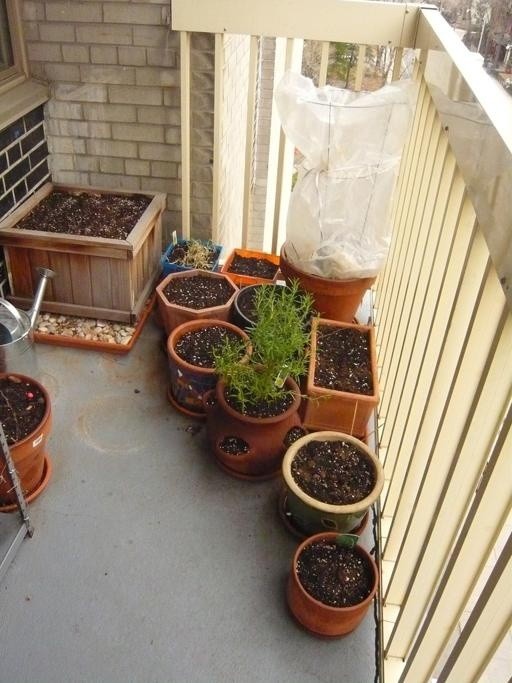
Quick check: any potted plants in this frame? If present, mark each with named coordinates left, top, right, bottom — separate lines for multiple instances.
left=285, top=531, right=378, bottom=640
left=282, top=431, right=385, bottom=541
left=161, top=239, right=379, bottom=483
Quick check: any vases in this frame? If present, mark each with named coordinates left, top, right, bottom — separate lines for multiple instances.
left=0, top=372, right=53, bottom=516
left=0, top=174, right=168, bottom=325
